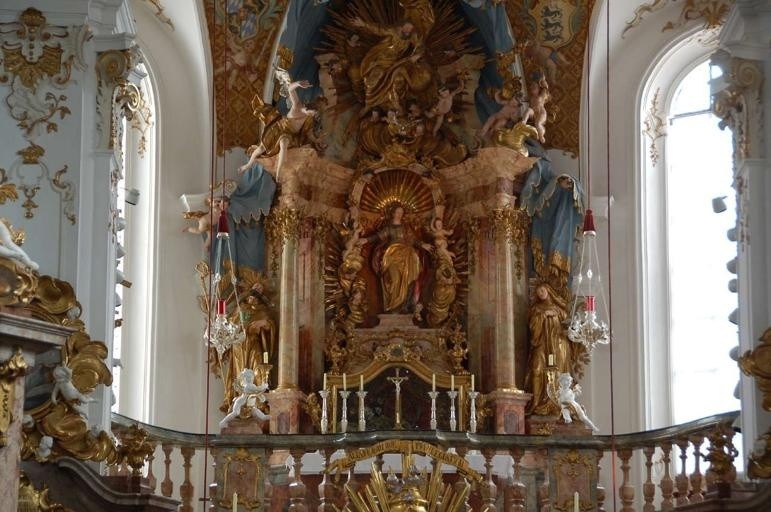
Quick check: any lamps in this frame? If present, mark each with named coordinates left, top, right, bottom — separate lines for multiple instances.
left=566, top=0, right=615, bottom=353
left=202, top=1, right=248, bottom=361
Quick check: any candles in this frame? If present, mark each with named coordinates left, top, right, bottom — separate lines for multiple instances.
left=432, top=371, right=476, bottom=391
left=322, top=371, right=365, bottom=391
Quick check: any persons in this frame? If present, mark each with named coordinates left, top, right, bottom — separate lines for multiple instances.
left=48, top=362, right=98, bottom=420
left=1, top=222, right=41, bottom=271
left=34, top=435, right=53, bottom=459
left=182, top=17, right=601, bottom=432
